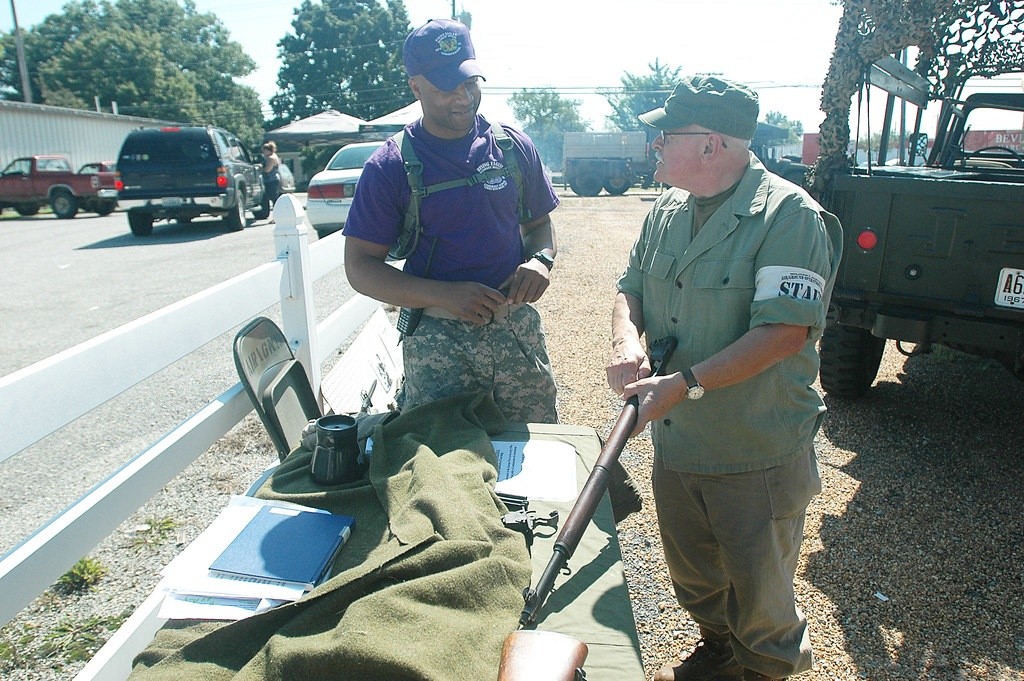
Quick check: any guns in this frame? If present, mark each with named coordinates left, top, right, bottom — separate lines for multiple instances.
left=520, top=336, right=679, bottom=627
left=501, top=504, right=558, bottom=559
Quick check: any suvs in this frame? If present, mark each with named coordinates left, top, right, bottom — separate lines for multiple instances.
left=110, top=122, right=268, bottom=237
left=818, top=90, right=1023, bottom=416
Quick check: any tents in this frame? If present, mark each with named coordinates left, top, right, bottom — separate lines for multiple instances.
left=357, top=96, right=427, bottom=142
left=261, top=109, right=368, bottom=149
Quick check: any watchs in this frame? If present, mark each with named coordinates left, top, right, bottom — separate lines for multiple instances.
left=679, top=367, right=705, bottom=400
left=527, top=252, right=555, bottom=272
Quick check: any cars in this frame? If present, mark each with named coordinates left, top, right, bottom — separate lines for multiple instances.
left=301, top=140, right=395, bottom=238
left=254, top=163, right=294, bottom=198
left=76, top=161, right=118, bottom=175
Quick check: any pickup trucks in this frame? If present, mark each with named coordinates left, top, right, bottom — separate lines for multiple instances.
left=0, top=155, right=120, bottom=219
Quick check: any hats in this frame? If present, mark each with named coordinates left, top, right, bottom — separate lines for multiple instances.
left=637, top=74, right=760, bottom=141
left=402, top=19, right=486, bottom=93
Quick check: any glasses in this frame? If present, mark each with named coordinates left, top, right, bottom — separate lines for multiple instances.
left=659, top=128, right=727, bottom=148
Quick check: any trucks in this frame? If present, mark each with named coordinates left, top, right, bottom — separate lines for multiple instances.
left=560, top=124, right=665, bottom=196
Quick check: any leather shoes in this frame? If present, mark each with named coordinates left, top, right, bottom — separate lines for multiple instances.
left=743, top=667, right=786, bottom=681
left=653, top=635, right=744, bottom=681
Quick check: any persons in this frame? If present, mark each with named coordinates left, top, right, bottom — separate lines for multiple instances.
left=338, top=19, right=560, bottom=426
left=604, top=74, right=845, bottom=681
left=254, top=140, right=282, bottom=220
